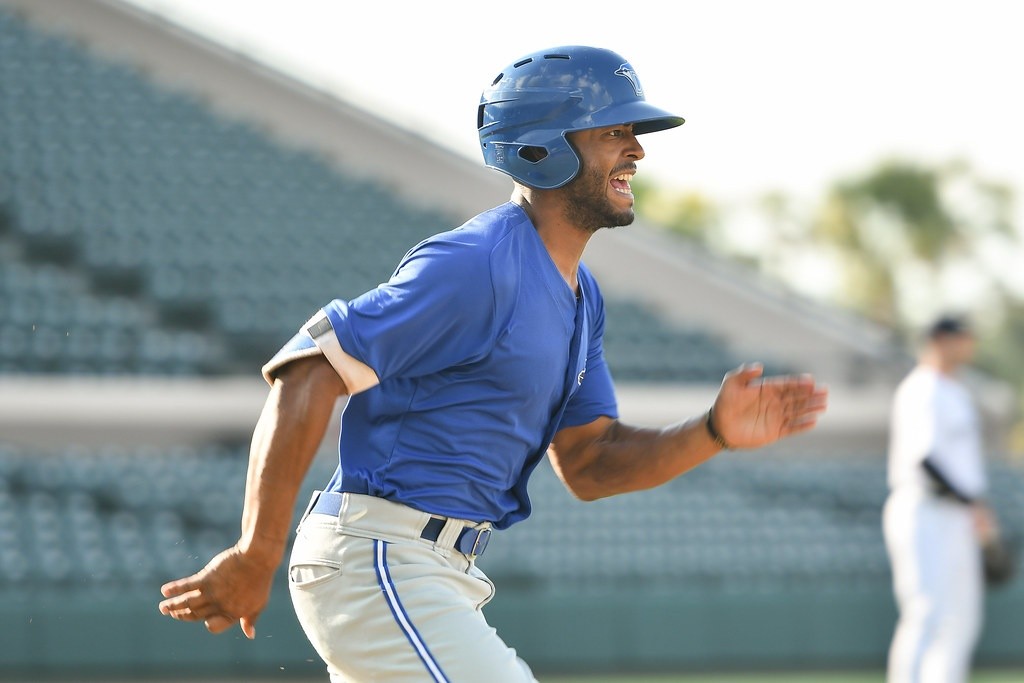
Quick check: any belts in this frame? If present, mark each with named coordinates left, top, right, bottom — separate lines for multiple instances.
left=309, top=492, right=491, bottom=557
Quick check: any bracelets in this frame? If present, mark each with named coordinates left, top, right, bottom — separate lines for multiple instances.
left=706, top=406, right=733, bottom=454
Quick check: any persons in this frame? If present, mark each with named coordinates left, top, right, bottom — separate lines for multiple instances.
left=158, top=45, right=830, bottom=682
left=880, top=316, right=1000, bottom=683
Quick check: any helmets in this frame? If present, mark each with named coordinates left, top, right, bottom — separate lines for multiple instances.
left=477, top=45, right=686, bottom=190
